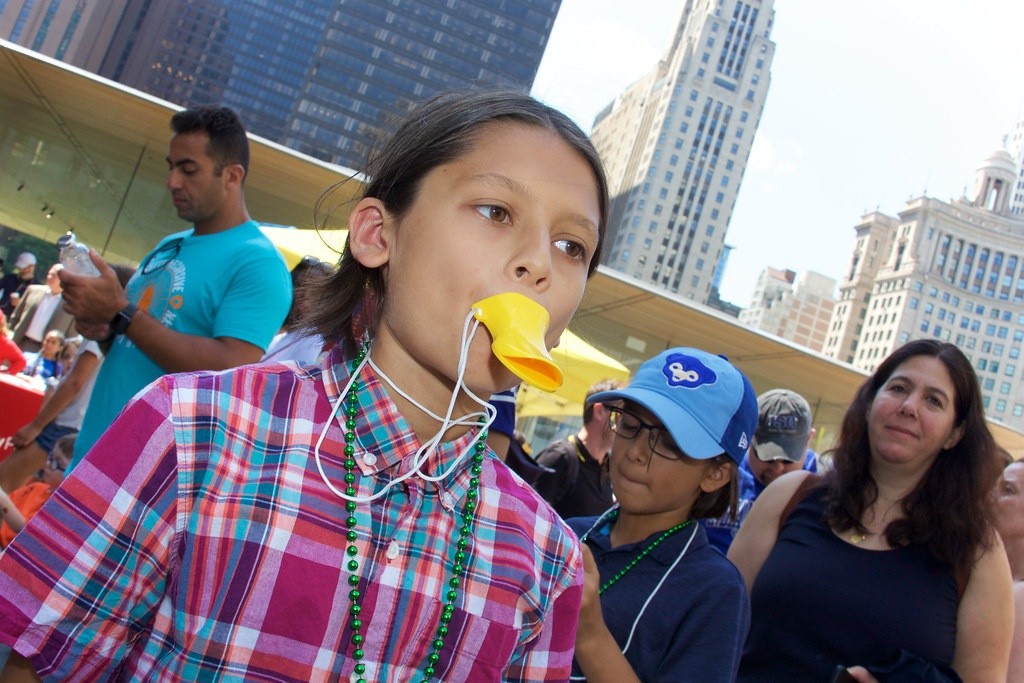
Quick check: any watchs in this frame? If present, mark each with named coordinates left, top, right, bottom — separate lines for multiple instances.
left=110, top=303, right=138, bottom=334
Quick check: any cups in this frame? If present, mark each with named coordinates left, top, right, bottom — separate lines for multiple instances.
left=10, top=292, right=19, bottom=306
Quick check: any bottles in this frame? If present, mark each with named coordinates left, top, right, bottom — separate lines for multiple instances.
left=58, top=234, right=102, bottom=277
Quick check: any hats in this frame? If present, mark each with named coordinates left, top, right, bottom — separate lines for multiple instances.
left=14, top=252, right=36, bottom=269
left=751, top=388, right=813, bottom=463
left=586, top=347, right=759, bottom=467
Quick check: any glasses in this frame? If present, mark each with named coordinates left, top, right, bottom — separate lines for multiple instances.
left=46, top=452, right=66, bottom=473
left=752, top=446, right=794, bottom=465
left=610, top=403, right=725, bottom=464
left=300, top=255, right=335, bottom=275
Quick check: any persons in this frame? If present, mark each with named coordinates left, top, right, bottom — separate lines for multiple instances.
left=0, top=88, right=610, bottom=683
left=726, top=339, right=1014, bottom=683
left=58, top=104, right=293, bottom=477
left=564, top=347, right=759, bottom=683
left=0, top=253, right=833, bottom=553
left=988, top=458, right=1024, bottom=683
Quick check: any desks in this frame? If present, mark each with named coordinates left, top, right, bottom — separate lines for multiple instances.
left=0, top=364, right=47, bottom=465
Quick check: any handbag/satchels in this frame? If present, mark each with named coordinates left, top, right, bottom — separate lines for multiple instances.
left=504, top=435, right=556, bottom=487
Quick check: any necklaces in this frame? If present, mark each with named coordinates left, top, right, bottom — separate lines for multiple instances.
left=345, top=349, right=488, bottom=683
left=581, top=510, right=691, bottom=594
left=850, top=531, right=867, bottom=543
